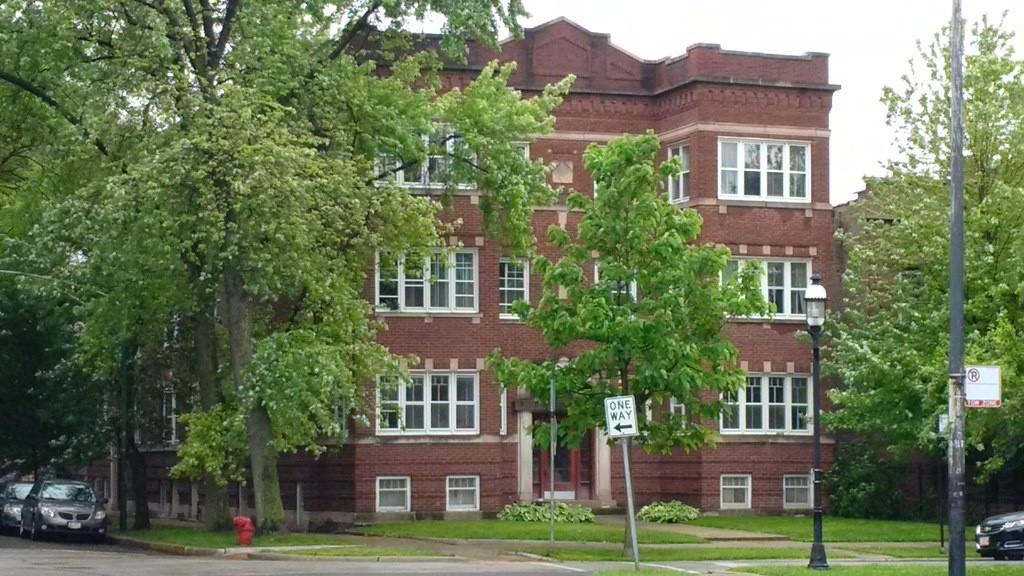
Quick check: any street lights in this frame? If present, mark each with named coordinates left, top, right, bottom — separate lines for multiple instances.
left=801, top=272, right=831, bottom=570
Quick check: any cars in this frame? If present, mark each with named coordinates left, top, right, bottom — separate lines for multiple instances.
left=19, top=477, right=109, bottom=544
left=975, top=510, right=1024, bottom=561
left=0, top=480, right=37, bottom=536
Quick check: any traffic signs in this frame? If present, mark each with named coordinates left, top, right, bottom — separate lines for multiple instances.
left=605, top=393, right=639, bottom=437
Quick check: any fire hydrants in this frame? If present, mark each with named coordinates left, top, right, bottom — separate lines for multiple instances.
left=233, top=515, right=255, bottom=547
